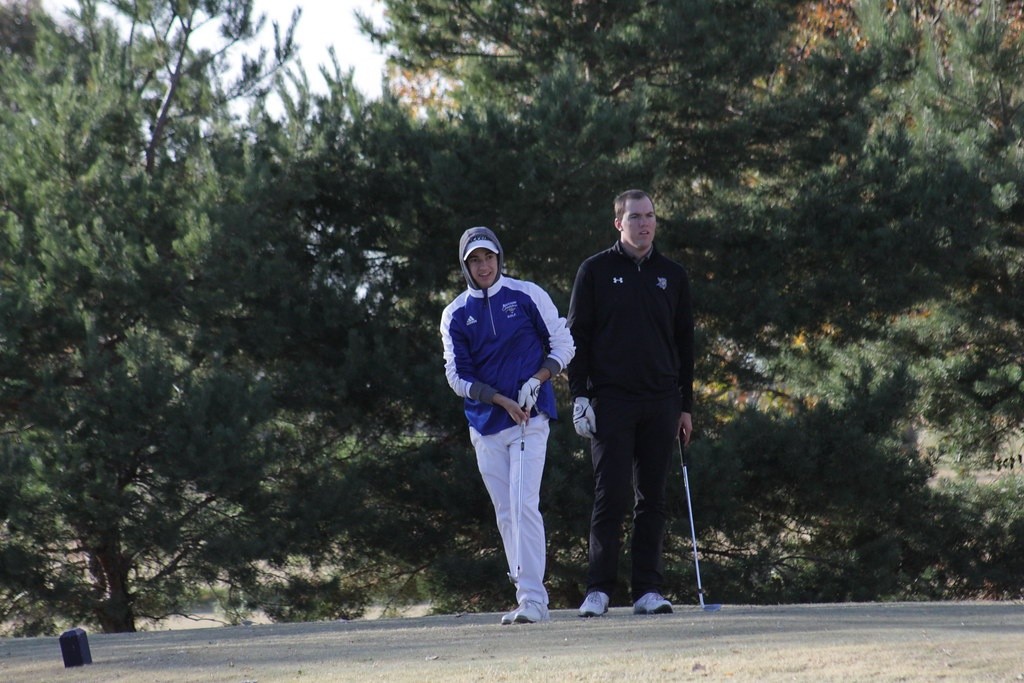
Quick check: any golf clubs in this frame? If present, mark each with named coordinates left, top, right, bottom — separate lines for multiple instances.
left=676, top=424, right=723, bottom=614
left=506, top=390, right=526, bottom=586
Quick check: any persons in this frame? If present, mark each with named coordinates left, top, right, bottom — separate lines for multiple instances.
left=438, top=225, right=578, bottom=625
left=563, top=188, right=696, bottom=617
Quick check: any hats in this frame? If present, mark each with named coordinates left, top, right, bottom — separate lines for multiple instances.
left=462, top=236, right=500, bottom=261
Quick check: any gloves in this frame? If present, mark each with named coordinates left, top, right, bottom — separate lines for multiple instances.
left=517, top=378, right=543, bottom=415
left=572, top=398, right=598, bottom=440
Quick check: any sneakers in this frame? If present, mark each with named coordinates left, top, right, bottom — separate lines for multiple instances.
left=500, top=600, right=552, bottom=625
left=632, top=592, right=674, bottom=615
left=577, top=591, right=610, bottom=617
